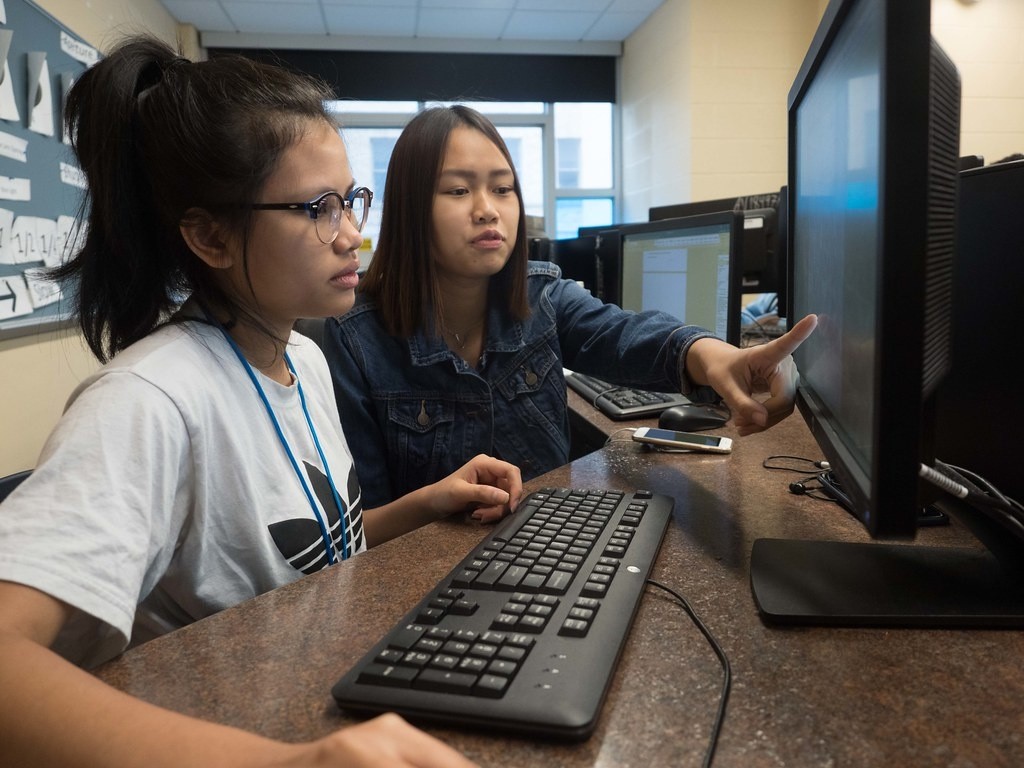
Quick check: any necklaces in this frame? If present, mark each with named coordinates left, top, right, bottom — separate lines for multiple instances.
left=445, top=317, right=488, bottom=350
left=193, top=290, right=346, bottom=567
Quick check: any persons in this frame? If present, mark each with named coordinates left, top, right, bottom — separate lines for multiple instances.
left=319, top=105, right=819, bottom=509
left=0, top=37, right=524, bottom=768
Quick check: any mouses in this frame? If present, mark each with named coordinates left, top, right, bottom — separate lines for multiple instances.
left=657, top=405, right=726, bottom=431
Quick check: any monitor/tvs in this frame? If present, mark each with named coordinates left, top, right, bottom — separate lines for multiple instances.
left=527, top=0, right=1024, bottom=629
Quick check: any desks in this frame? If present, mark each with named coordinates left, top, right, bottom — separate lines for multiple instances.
left=86, top=375, right=1024, bottom=768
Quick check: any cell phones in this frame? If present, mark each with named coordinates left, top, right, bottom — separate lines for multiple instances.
left=631, top=426, right=734, bottom=453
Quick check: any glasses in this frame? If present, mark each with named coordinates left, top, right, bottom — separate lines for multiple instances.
left=211, top=187, right=373, bottom=244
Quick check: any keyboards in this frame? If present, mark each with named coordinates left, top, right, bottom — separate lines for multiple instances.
left=566, top=372, right=695, bottom=419
left=331, top=488, right=675, bottom=739
